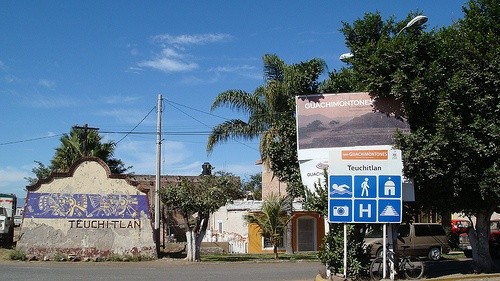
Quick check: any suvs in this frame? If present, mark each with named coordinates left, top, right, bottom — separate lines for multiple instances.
left=458, top=219, right=500, bottom=259
left=360, top=223, right=450, bottom=263
left=13, top=215, right=22, bottom=226
left=450, top=218, right=476, bottom=238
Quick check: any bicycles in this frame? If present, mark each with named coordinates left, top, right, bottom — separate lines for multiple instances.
left=369, top=242, right=425, bottom=281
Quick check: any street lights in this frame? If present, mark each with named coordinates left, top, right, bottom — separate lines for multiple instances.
left=338, top=15, right=429, bottom=281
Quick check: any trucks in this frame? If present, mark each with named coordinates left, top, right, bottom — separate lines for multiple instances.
left=0, top=194, right=17, bottom=249
